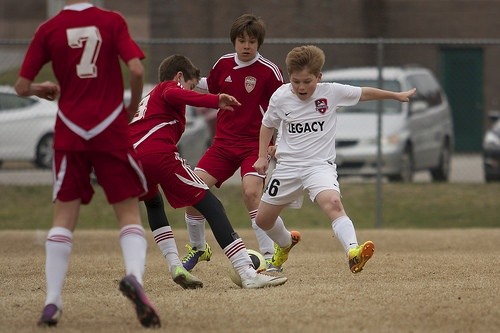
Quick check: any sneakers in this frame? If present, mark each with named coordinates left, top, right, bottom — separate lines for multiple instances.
left=262, top=252, right=273, bottom=269
left=348, top=239, right=376, bottom=274
left=271, top=230, right=302, bottom=268
left=170, top=265, right=204, bottom=290
left=117, top=273, right=161, bottom=328
left=36, top=304, right=62, bottom=327
left=181, top=243, right=212, bottom=273
left=241, top=272, right=288, bottom=290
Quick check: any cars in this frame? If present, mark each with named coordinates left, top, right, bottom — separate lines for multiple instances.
left=480, top=113, right=500, bottom=182
left=0, top=86, right=58, bottom=169
left=90, top=77, right=221, bottom=184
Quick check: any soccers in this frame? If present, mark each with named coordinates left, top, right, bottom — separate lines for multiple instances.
left=228, top=249, right=267, bottom=287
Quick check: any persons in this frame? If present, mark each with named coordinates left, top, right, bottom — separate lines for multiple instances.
left=253, top=46, right=416, bottom=273
left=181, top=13, right=286, bottom=271
left=127, top=53, right=288, bottom=289
left=16, top=0, right=162, bottom=330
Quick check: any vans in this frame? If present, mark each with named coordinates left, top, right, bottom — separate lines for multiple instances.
left=317, top=65, right=454, bottom=183
